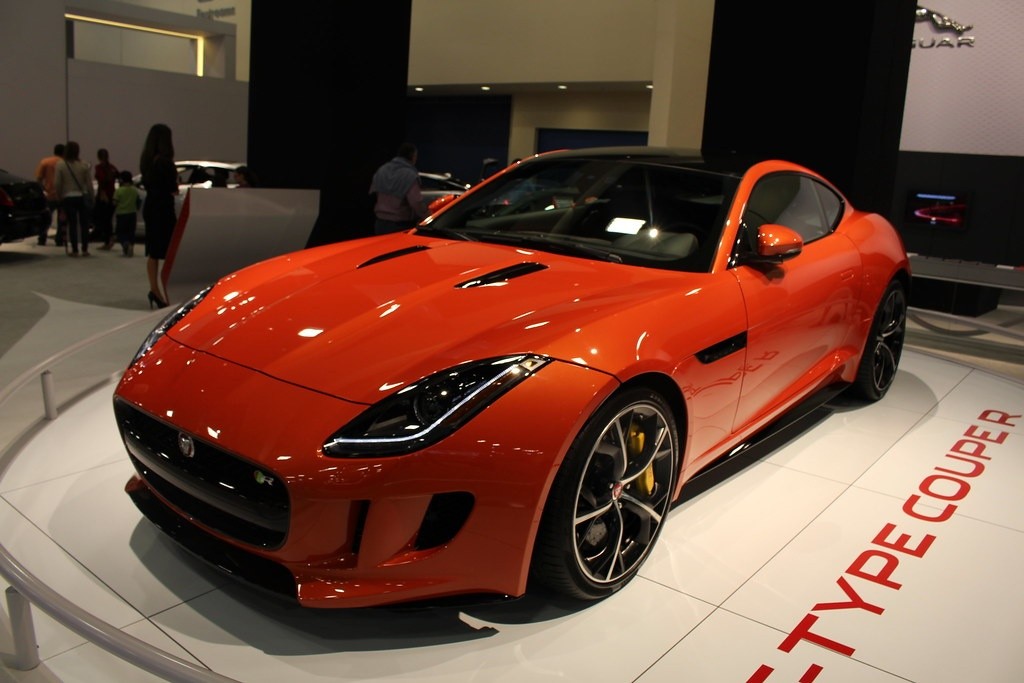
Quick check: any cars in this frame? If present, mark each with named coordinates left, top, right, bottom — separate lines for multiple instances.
left=0, top=168, right=53, bottom=244
left=417, top=171, right=470, bottom=216
left=50, top=160, right=247, bottom=240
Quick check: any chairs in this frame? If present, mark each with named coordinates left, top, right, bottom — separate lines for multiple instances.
left=721, top=190, right=782, bottom=226
left=551, top=185, right=656, bottom=241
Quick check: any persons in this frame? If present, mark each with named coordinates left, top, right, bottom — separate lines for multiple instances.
left=114, top=171, right=142, bottom=255
left=234, top=167, right=251, bottom=187
left=95, top=149, right=119, bottom=250
left=482, top=158, right=536, bottom=192
left=373, top=142, right=428, bottom=234
left=55, top=142, right=94, bottom=256
left=35, top=144, right=64, bottom=247
left=140, top=123, right=178, bottom=309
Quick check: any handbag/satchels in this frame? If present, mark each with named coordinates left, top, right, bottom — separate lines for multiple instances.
left=85, top=193, right=95, bottom=208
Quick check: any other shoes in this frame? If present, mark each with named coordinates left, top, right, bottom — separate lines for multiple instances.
left=82, top=252, right=90, bottom=257
left=102, top=242, right=113, bottom=250
left=127, top=245, right=133, bottom=257
left=69, top=252, right=78, bottom=257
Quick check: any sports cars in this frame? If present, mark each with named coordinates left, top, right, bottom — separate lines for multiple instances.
left=112, top=145, right=912, bottom=610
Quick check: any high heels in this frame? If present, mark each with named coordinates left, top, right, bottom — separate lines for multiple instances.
left=148, top=291, right=167, bottom=308
